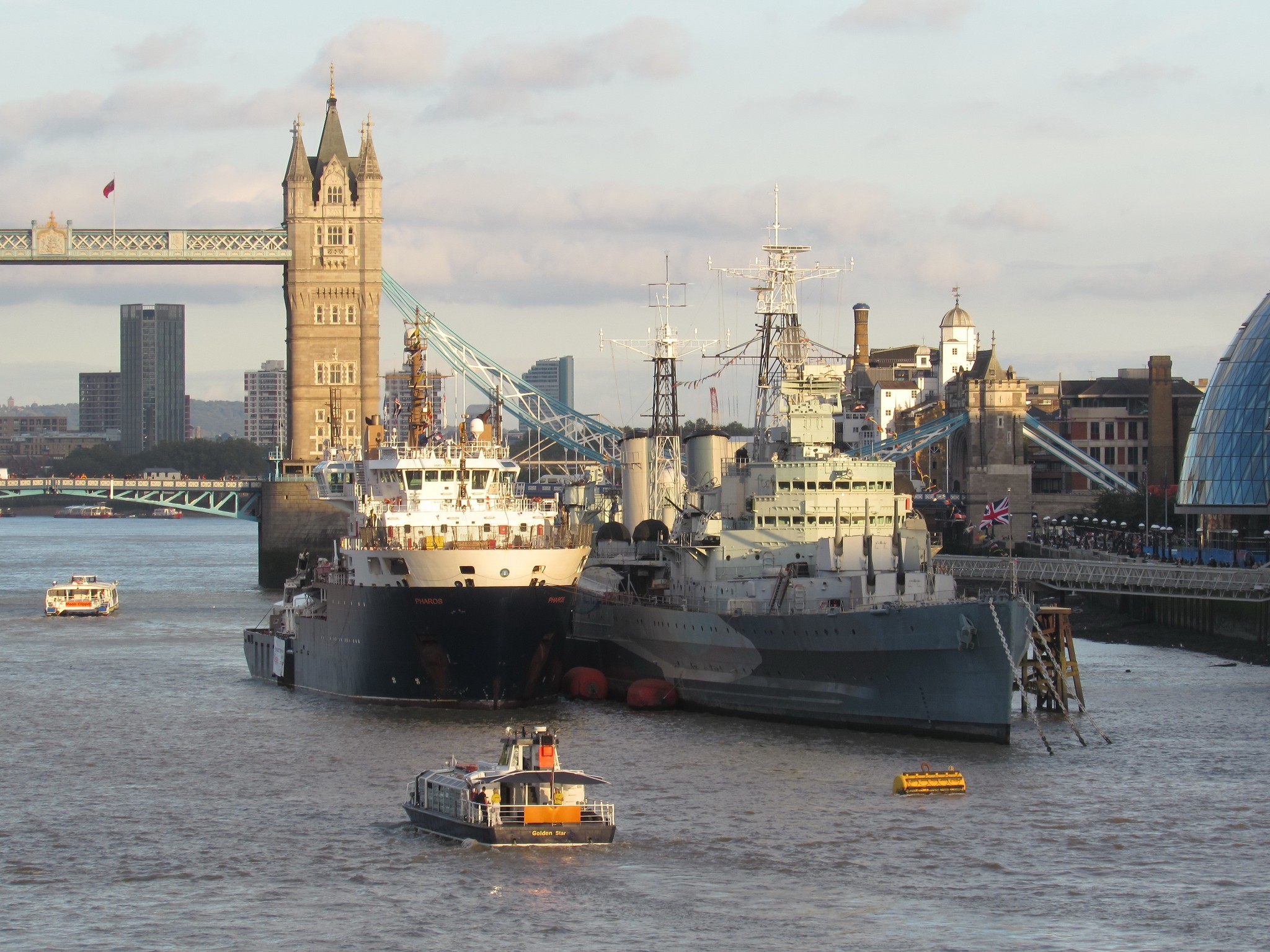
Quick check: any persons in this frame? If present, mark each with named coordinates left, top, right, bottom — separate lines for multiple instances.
left=8, top=473, right=17, bottom=478
left=553, top=788, right=564, bottom=825
left=472, top=787, right=503, bottom=826
left=172, top=475, right=258, bottom=482
left=69, top=472, right=154, bottom=480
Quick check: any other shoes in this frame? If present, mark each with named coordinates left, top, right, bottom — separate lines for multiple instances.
left=483, top=822, right=487, bottom=824
left=552, top=824, right=557, bottom=825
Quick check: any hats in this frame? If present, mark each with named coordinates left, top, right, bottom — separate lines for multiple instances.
left=472, top=788, right=476, bottom=793
left=492, top=788, right=499, bottom=793
left=555, top=787, right=560, bottom=790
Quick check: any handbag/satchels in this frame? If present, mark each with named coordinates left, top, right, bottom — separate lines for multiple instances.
left=490, top=809, right=495, bottom=813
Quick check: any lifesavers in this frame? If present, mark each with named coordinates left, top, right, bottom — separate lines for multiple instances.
left=820, top=601, right=827, bottom=609
left=465, top=764, right=477, bottom=773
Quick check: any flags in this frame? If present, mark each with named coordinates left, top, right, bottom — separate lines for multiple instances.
left=671, top=337, right=1013, bottom=558
left=383, top=393, right=447, bottom=432
left=103, top=178, right=115, bottom=198
left=550, top=766, right=554, bottom=800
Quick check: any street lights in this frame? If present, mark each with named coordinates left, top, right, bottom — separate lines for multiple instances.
left=1101, top=519, right=1109, bottom=551
left=1160, top=526, right=1171, bottom=565
left=1033, top=516, right=1080, bottom=546
left=1120, top=522, right=1131, bottom=557
left=1231, top=530, right=1241, bottom=568
left=1263, top=530, right=1270, bottom=564
left=1093, top=518, right=1100, bottom=549
left=1008, top=514, right=1014, bottom=541
left=1151, top=524, right=1164, bottom=561
left=1196, top=528, right=1205, bottom=566
left=1139, top=523, right=1150, bottom=558
left=1166, top=527, right=1175, bottom=563
left=1083, top=517, right=1090, bottom=538
left=1110, top=520, right=1118, bottom=552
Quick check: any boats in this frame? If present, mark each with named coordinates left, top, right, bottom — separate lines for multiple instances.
left=44, top=575, right=118, bottom=617
left=240, top=185, right=1039, bottom=744
left=126, top=514, right=139, bottom=519
left=138, top=513, right=149, bottom=519
left=413, top=726, right=628, bottom=848
left=112, top=513, right=128, bottom=518
left=54, top=501, right=114, bottom=519
left=150, top=506, right=184, bottom=519
left=0, top=508, right=16, bottom=517
